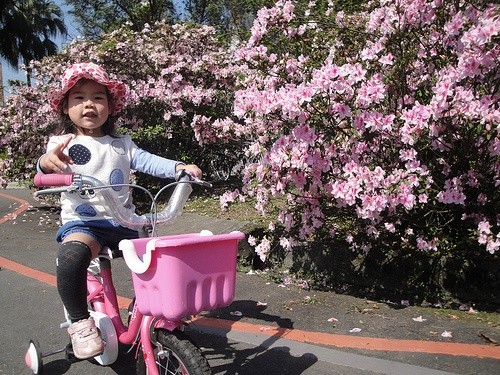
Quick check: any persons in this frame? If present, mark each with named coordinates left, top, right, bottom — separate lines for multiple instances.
left=37, top=63, right=202, bottom=360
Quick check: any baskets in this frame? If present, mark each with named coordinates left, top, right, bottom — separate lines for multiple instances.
left=118, top=231, right=246, bottom=320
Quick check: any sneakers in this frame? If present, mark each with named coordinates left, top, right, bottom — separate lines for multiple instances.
left=66, top=316, right=104, bottom=359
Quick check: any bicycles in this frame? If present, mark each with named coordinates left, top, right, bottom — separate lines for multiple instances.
left=22, top=173, right=245, bottom=375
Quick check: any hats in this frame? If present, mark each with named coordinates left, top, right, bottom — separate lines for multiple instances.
left=48, top=62, right=127, bottom=117
